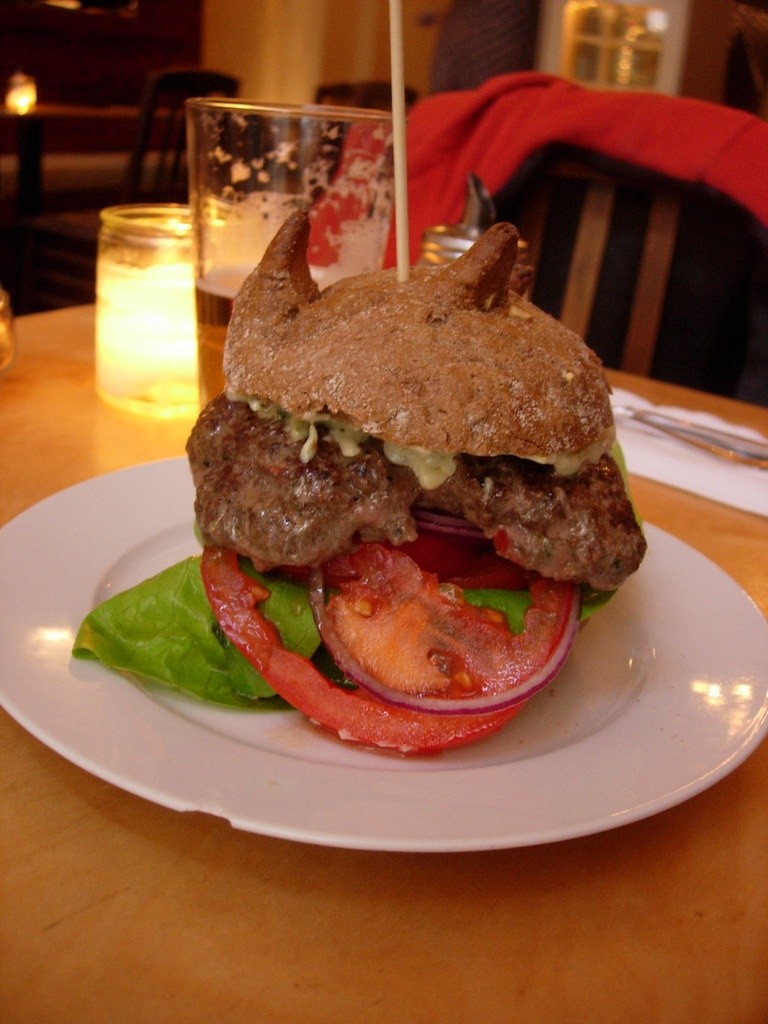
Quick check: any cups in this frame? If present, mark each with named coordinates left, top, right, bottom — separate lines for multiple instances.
left=184, top=96, right=410, bottom=409
left=94, top=204, right=199, bottom=420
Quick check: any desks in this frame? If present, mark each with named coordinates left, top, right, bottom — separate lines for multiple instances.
left=0, top=149, right=190, bottom=200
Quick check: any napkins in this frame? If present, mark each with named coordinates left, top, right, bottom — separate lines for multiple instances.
left=608, top=385, right=767, bottom=516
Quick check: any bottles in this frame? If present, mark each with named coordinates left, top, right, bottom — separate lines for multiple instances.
left=423, top=174, right=500, bottom=273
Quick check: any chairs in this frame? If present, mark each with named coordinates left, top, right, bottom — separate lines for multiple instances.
left=484, top=142, right=684, bottom=378
left=20, top=66, right=240, bottom=315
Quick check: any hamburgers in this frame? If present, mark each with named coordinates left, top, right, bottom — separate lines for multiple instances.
left=69, top=212, right=648, bottom=754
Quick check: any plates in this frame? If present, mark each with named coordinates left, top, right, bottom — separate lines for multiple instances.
left=0, top=457, right=768, bottom=853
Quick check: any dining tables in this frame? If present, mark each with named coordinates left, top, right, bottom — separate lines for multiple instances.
left=0, top=303, right=767, bottom=1024
